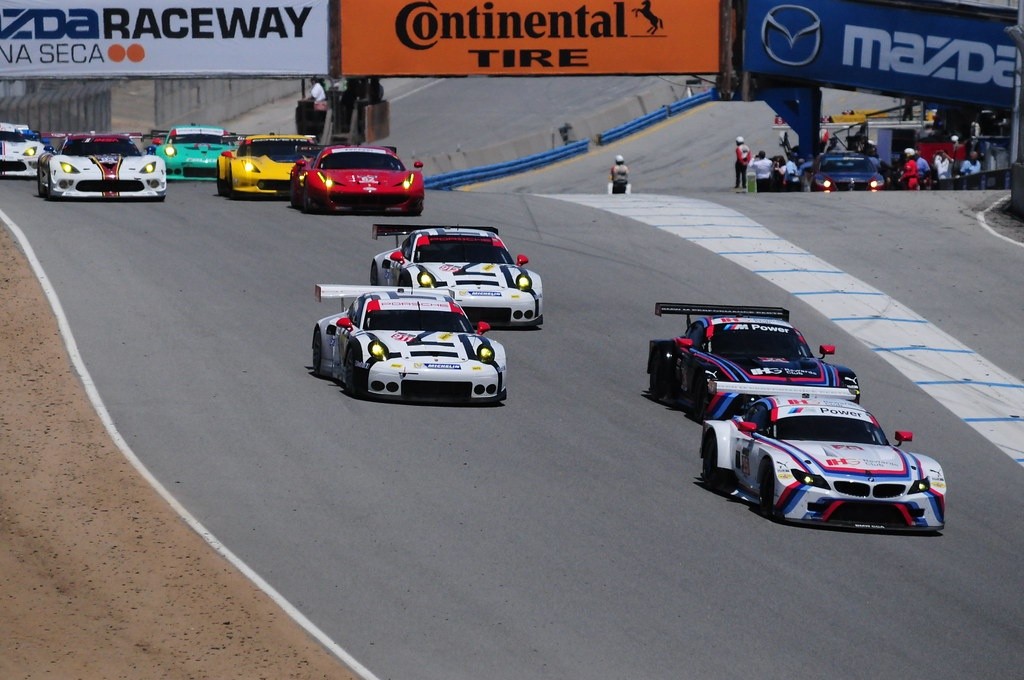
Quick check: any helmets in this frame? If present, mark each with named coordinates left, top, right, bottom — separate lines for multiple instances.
left=735, top=136, right=744, bottom=143
left=904, top=148, right=916, bottom=156
left=615, top=155, right=624, bottom=162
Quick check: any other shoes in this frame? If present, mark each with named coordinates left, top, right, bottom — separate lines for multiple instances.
left=742, top=188, right=747, bottom=193
left=734, top=188, right=741, bottom=193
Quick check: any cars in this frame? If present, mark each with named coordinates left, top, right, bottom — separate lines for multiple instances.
left=810, top=151, right=885, bottom=190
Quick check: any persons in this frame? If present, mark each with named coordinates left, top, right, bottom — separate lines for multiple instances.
left=610, top=155, right=629, bottom=194
left=878, top=146, right=981, bottom=191
left=343, top=78, right=384, bottom=106
left=735, top=136, right=751, bottom=188
left=303, top=78, right=327, bottom=101
left=748, top=151, right=813, bottom=193
left=902, top=98, right=913, bottom=121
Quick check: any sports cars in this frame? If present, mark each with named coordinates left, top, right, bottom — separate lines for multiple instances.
left=312, top=283, right=507, bottom=404
left=0, top=123, right=57, bottom=177
left=698, top=381, right=947, bottom=532
left=37, top=131, right=167, bottom=202
left=289, top=144, right=425, bottom=215
left=647, top=302, right=860, bottom=424
left=370, top=223, right=543, bottom=327
left=217, top=134, right=318, bottom=200
left=145, top=122, right=239, bottom=181
